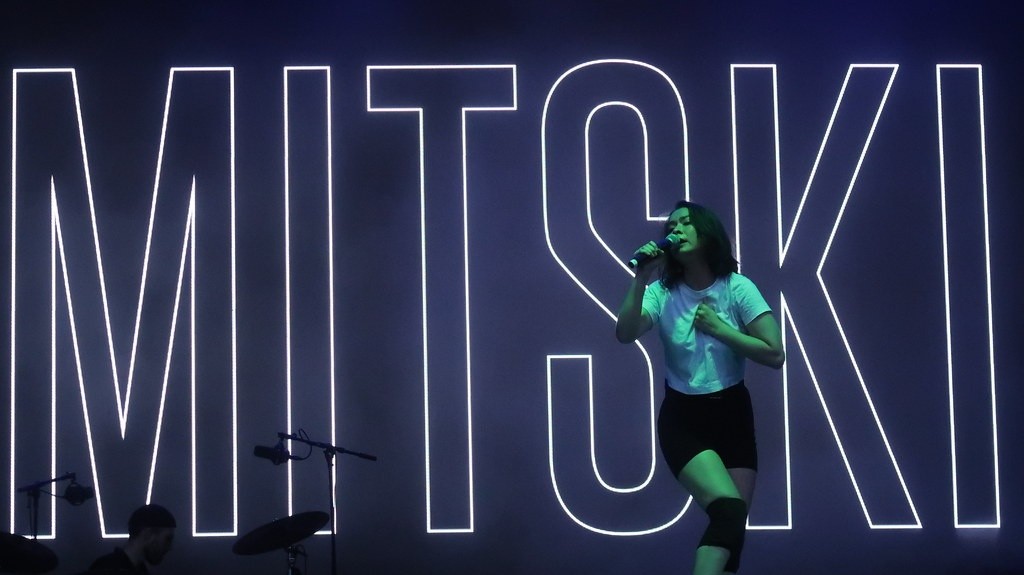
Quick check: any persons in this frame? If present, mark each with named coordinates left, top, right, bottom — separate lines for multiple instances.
left=82, top=503, right=178, bottom=575
left=614, top=200, right=786, bottom=575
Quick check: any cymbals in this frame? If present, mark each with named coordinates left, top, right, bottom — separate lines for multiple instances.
left=0, top=530, right=59, bottom=574
left=232, top=510, right=330, bottom=556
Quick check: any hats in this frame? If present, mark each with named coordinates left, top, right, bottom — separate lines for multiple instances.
left=128, top=504, right=176, bottom=528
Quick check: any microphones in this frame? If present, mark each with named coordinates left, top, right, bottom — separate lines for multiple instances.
left=65, top=486, right=95, bottom=501
left=628, top=232, right=681, bottom=269
left=252, top=444, right=289, bottom=465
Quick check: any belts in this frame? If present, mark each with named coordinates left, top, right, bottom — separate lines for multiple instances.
left=666, top=383, right=746, bottom=403
